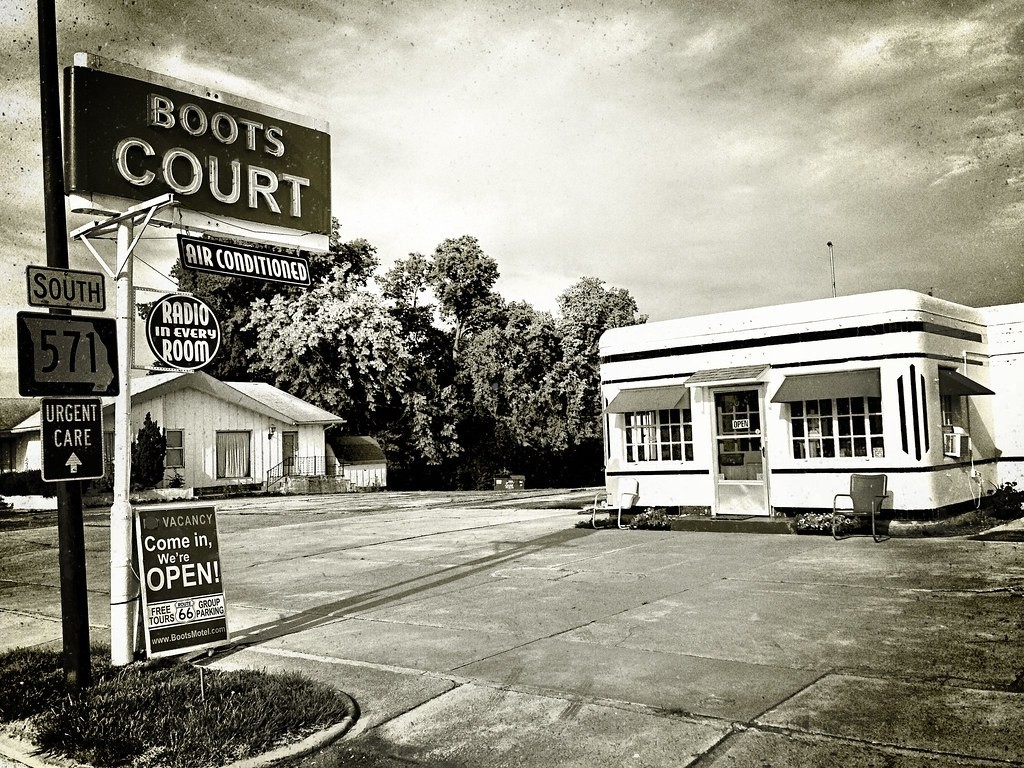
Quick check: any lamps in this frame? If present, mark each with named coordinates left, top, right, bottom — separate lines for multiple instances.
left=268, top=423, right=276, bottom=440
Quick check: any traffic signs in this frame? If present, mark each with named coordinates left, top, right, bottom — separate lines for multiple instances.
left=40, top=397, right=107, bottom=483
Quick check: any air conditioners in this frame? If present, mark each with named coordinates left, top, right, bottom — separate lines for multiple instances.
left=943, top=433, right=969, bottom=458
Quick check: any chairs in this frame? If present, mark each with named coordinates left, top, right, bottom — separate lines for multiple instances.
left=832, top=474, right=890, bottom=544
left=592, top=477, right=639, bottom=529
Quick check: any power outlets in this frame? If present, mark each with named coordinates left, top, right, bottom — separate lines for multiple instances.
left=971, top=470, right=981, bottom=477
